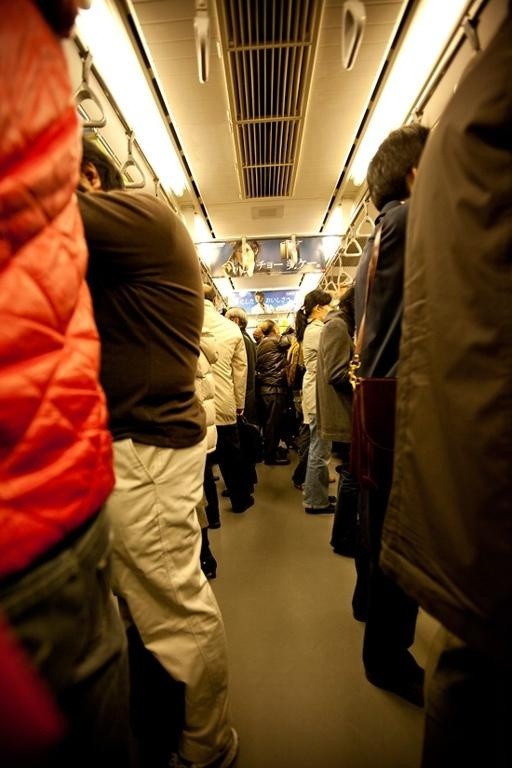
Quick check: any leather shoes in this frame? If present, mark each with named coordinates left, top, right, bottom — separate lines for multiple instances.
left=305, top=496, right=337, bottom=514
left=265, top=458, right=289, bottom=465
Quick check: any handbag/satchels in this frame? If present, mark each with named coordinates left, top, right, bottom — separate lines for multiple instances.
left=350, top=378, right=394, bottom=486
left=293, top=364, right=305, bottom=390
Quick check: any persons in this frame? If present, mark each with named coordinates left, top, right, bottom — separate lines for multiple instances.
left=352, top=124, right=430, bottom=706
left=194, top=284, right=258, bottom=579
left=0, top=2, right=128, bottom=767
left=380, top=21, right=512, bottom=768
left=79, top=138, right=239, bottom=768
left=295, top=286, right=374, bottom=619
left=252, top=320, right=303, bottom=465
